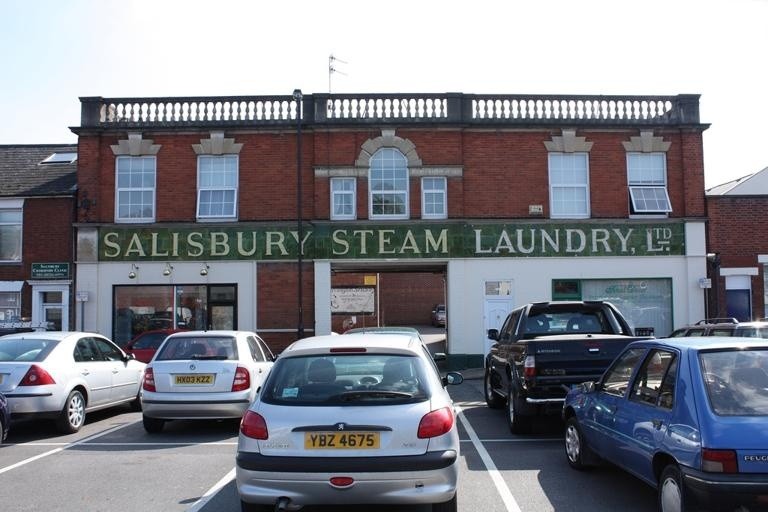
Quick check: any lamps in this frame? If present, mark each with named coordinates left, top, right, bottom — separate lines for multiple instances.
left=163, top=262, right=174, bottom=275
left=199, top=262, right=209, bottom=275
left=128, top=262, right=139, bottom=279
left=684, top=253, right=717, bottom=263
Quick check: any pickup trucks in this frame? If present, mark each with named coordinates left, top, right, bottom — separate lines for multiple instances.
left=484, top=299, right=663, bottom=442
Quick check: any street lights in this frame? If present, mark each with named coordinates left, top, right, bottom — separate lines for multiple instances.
left=291, top=88, right=308, bottom=342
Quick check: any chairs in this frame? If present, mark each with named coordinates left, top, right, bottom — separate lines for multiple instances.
left=729, top=367, right=768, bottom=408
left=300, top=359, right=348, bottom=393
left=527, top=314, right=550, bottom=332
left=374, top=358, right=412, bottom=387
left=189, top=343, right=207, bottom=354
left=216, top=347, right=227, bottom=355
left=565, top=314, right=602, bottom=333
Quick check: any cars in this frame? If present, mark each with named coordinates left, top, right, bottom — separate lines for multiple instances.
left=234, top=332, right=465, bottom=512
left=431, top=303, right=446, bottom=327
left=331, top=326, right=446, bottom=394
left=0, top=329, right=149, bottom=435
left=559, top=317, right=768, bottom=512
left=140, top=330, right=278, bottom=434
left=118, top=306, right=190, bottom=363
